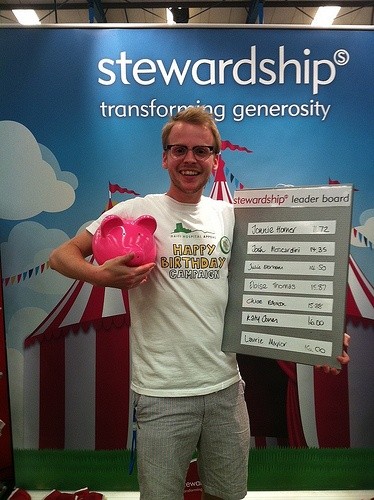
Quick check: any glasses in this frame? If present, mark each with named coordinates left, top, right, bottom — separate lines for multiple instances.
left=171, top=143, right=216, bottom=161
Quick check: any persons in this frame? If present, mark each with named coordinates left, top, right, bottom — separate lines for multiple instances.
left=48, top=107, right=350, bottom=500
